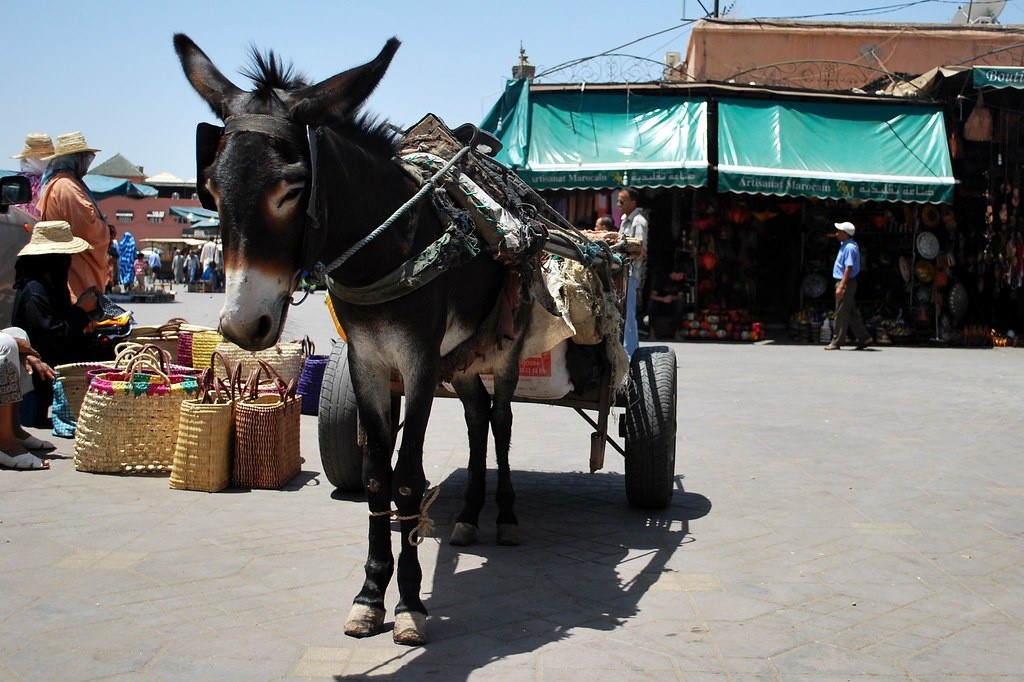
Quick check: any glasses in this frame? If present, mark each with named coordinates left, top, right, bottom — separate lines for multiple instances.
left=616, top=199, right=631, bottom=204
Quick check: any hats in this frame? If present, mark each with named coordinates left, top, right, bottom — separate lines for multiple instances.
left=834, top=222, right=855, bottom=236
left=17, top=221, right=89, bottom=257
left=40, top=131, right=102, bottom=160
left=10, top=133, right=55, bottom=158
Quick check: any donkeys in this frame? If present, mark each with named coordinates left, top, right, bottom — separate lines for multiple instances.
left=173, top=32, right=534, bottom=644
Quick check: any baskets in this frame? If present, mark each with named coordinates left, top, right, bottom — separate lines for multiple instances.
left=52, top=318, right=336, bottom=494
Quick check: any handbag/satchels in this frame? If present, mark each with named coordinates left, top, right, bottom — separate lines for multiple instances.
left=964, top=106, right=993, bottom=141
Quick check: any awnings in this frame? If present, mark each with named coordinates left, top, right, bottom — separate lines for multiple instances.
left=716, top=96, right=960, bottom=204
left=941, top=65, right=1024, bottom=90
left=518, top=89, right=709, bottom=191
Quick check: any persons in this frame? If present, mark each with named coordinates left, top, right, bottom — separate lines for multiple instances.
left=200, top=236, right=220, bottom=288
left=148, top=247, right=164, bottom=284
left=617, top=188, right=650, bottom=358
left=646, top=262, right=685, bottom=342
left=594, top=216, right=615, bottom=232
left=133, top=253, right=148, bottom=287
left=824, top=221, right=874, bottom=351
left=172, top=245, right=200, bottom=284
left=6, top=133, right=118, bottom=310
left=0, top=326, right=59, bottom=470
left=10, top=220, right=128, bottom=395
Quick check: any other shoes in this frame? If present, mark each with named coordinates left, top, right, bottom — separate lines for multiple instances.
left=824, top=344, right=840, bottom=350
left=855, top=338, right=874, bottom=350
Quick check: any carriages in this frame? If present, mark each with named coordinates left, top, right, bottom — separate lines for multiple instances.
left=172, top=30, right=677, bottom=647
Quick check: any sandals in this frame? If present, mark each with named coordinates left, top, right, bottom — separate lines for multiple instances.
left=16, top=435, right=55, bottom=450
left=0, top=451, right=50, bottom=469
